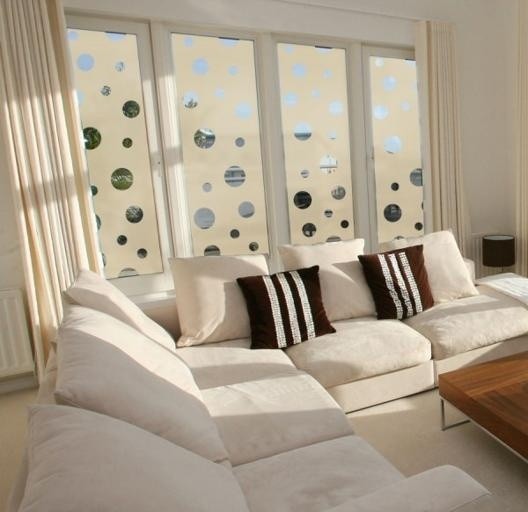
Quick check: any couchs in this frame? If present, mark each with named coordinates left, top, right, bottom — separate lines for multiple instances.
left=7, top=258, right=528, bottom=512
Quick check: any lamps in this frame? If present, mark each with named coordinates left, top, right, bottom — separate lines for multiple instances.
left=482, top=235, right=515, bottom=272
